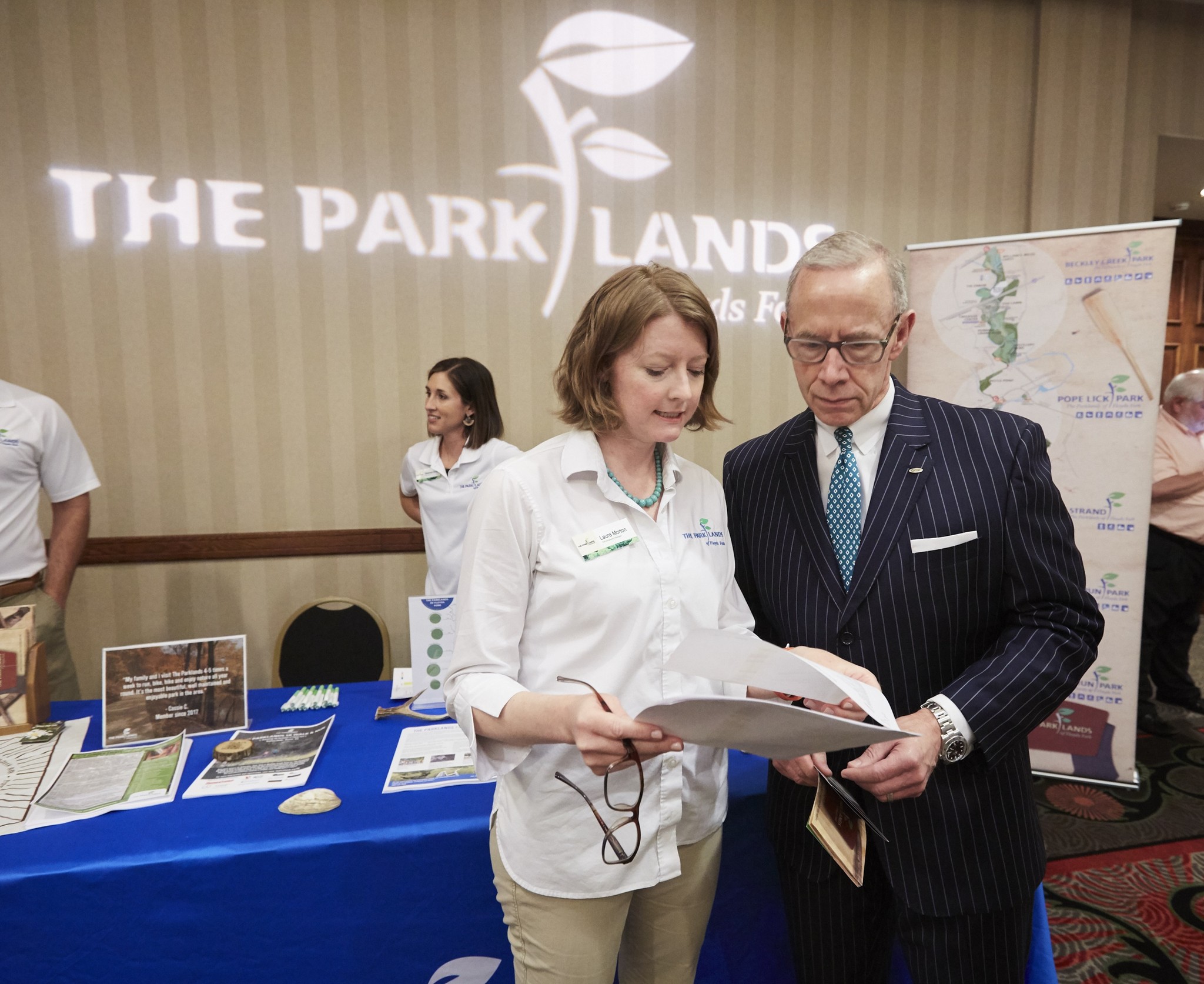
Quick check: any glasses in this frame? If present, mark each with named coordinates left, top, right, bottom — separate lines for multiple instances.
left=555, top=675, right=644, bottom=865
left=784, top=313, right=902, bottom=365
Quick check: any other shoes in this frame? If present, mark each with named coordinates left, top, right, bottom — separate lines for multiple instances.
left=1137, top=700, right=1179, bottom=740
left=1155, top=685, right=1204, bottom=714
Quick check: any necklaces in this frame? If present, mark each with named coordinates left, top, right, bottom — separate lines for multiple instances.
left=607, top=447, right=662, bottom=508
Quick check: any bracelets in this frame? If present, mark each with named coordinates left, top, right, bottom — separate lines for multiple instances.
left=774, top=647, right=804, bottom=701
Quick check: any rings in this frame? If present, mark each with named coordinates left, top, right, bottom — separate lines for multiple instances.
left=887, top=792, right=894, bottom=803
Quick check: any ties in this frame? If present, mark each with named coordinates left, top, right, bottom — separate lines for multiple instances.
left=825, top=426, right=862, bottom=595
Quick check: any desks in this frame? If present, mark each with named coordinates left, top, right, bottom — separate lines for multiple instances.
left=0, top=680, right=1062, bottom=984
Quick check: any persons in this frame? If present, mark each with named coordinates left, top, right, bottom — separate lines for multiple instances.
left=1141, top=368, right=1204, bottom=715
left=399, top=356, right=526, bottom=597
left=0, top=374, right=101, bottom=701
left=439, top=259, right=881, bottom=984
left=722, top=229, right=1106, bottom=984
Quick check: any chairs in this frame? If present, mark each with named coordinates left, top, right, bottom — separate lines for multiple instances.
left=269, top=596, right=393, bottom=688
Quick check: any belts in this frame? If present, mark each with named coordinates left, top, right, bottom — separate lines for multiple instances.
left=0, top=576, right=41, bottom=598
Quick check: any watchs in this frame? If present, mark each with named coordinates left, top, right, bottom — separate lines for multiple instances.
left=920, top=701, right=968, bottom=763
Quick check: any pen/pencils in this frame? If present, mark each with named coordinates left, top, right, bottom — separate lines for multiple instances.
left=281, top=684, right=339, bottom=712
